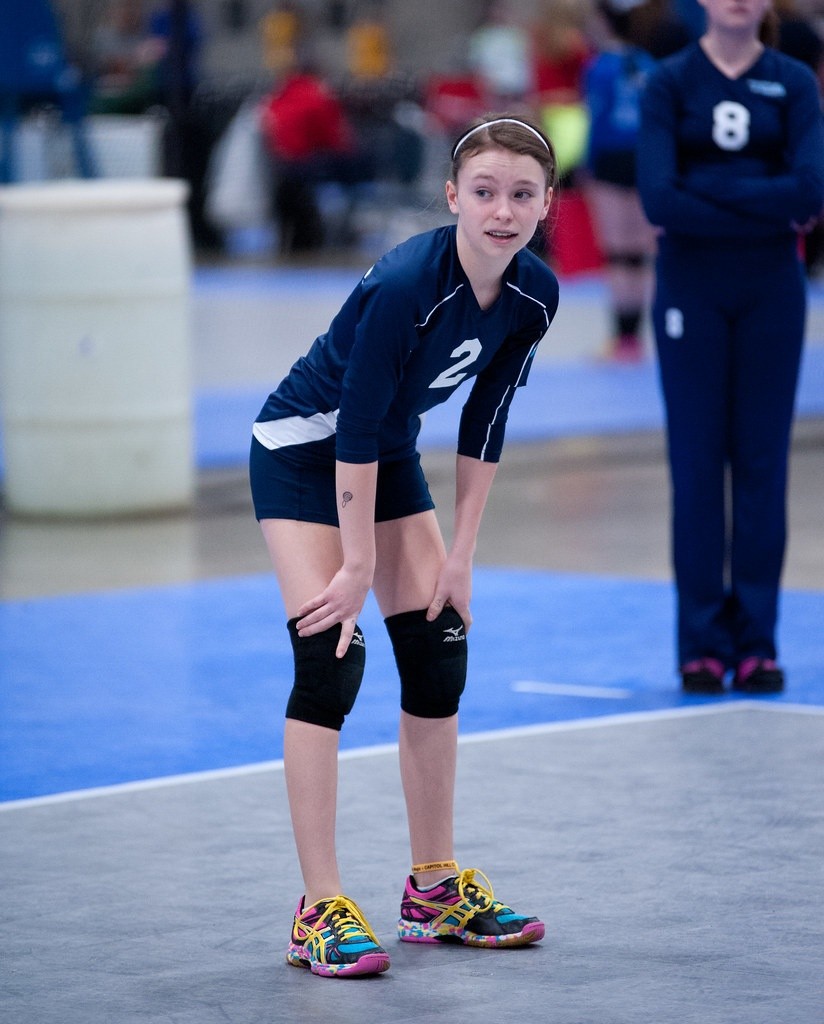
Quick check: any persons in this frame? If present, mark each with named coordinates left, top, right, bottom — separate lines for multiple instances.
left=640, top=0, right=824, bottom=696
left=467, top=0, right=540, bottom=103
left=0, top=0, right=98, bottom=185
left=561, top=0, right=665, bottom=361
left=135, top=0, right=253, bottom=258
left=759, top=0, right=824, bottom=278
left=341, top=0, right=393, bottom=77
left=248, top=117, right=560, bottom=977
left=258, top=0, right=299, bottom=76
left=264, top=55, right=349, bottom=257
left=428, top=64, right=484, bottom=132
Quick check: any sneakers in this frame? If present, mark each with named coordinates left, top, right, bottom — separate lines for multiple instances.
left=287, top=894, right=390, bottom=976
left=397, top=869, right=545, bottom=948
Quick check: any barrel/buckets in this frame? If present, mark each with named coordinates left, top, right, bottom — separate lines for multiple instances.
left=1, top=179, right=200, bottom=515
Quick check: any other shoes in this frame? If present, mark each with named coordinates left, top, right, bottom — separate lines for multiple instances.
left=733, top=657, right=784, bottom=694
left=603, top=336, right=642, bottom=368
left=683, top=659, right=725, bottom=696
